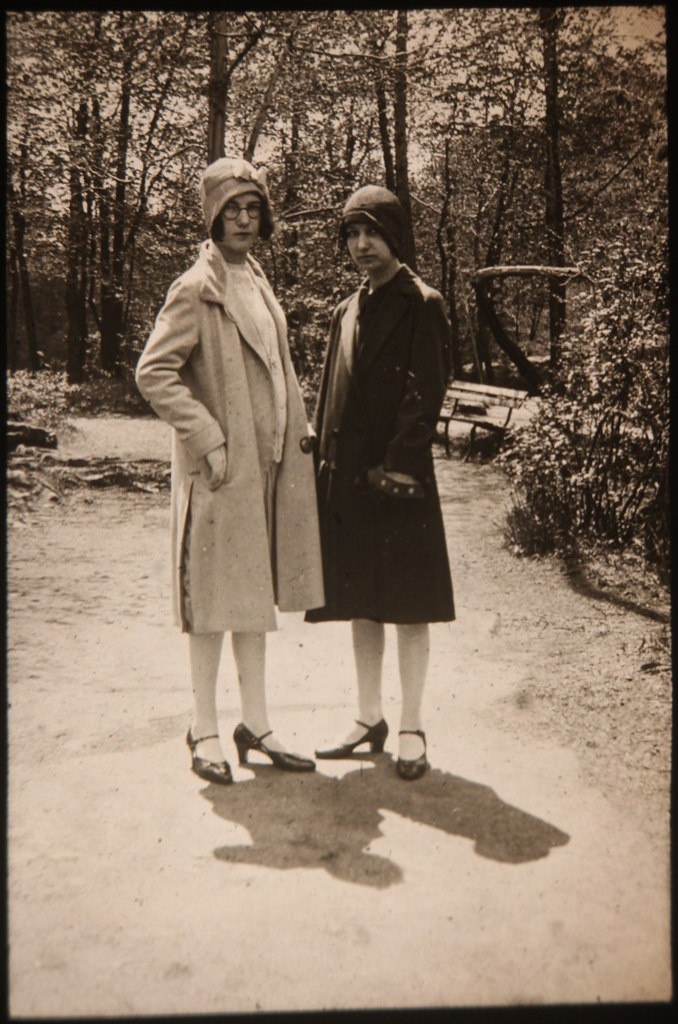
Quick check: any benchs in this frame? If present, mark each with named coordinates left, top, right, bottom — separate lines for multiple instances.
left=433, top=379, right=529, bottom=463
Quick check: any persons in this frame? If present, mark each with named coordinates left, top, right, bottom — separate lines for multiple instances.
left=304, top=184, right=453, bottom=782
left=133, top=157, right=325, bottom=786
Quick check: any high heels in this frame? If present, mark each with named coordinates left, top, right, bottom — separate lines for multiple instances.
left=187, top=727, right=233, bottom=784
left=234, top=723, right=317, bottom=771
left=315, top=716, right=389, bottom=758
left=398, top=728, right=426, bottom=779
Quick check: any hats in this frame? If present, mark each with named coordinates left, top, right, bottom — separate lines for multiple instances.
left=201, top=158, right=275, bottom=241
left=336, top=185, right=407, bottom=265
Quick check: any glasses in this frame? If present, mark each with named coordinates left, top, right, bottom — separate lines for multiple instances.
left=223, top=205, right=264, bottom=219
